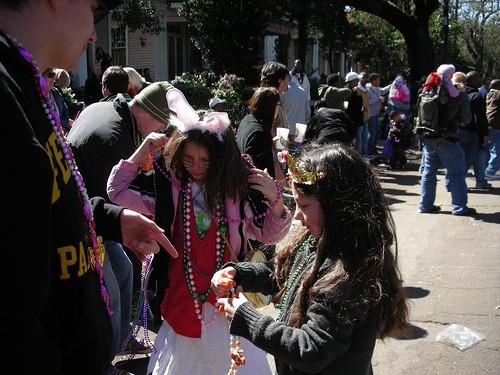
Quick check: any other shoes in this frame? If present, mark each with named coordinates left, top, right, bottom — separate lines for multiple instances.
left=368, top=153, right=494, bottom=216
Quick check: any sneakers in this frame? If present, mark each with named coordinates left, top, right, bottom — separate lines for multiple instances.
left=115, top=337, right=154, bottom=355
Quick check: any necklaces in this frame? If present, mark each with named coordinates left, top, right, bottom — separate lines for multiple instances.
left=213, top=276, right=247, bottom=375
left=0, top=31, right=113, bottom=317
left=120, top=253, right=158, bottom=365
left=149, top=137, right=283, bottom=328
left=276, top=234, right=316, bottom=325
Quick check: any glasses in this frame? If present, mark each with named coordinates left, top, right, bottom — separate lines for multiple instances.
left=43, top=72, right=55, bottom=78
left=275, top=103, right=282, bottom=107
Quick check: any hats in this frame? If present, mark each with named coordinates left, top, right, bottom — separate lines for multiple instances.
left=345, top=72, right=359, bottom=82
left=209, top=98, right=226, bottom=106
left=133, top=81, right=172, bottom=125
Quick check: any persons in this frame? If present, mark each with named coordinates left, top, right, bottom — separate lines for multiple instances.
left=432, top=63, right=463, bottom=141
left=210, top=142, right=411, bottom=375
left=235, top=87, right=284, bottom=265
left=289, top=59, right=311, bottom=108
left=35, top=44, right=156, bottom=183
left=417, top=72, right=477, bottom=215
left=0, top=0, right=181, bottom=374
left=483, top=78, right=500, bottom=182
left=279, top=70, right=311, bottom=157
left=256, top=60, right=292, bottom=176
left=67, top=81, right=180, bottom=375
left=444, top=69, right=493, bottom=192
left=104, top=88, right=293, bottom=375
left=303, top=66, right=427, bottom=170
left=477, top=72, right=495, bottom=98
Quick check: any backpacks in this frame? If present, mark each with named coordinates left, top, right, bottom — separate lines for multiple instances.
left=416, top=79, right=449, bottom=138
